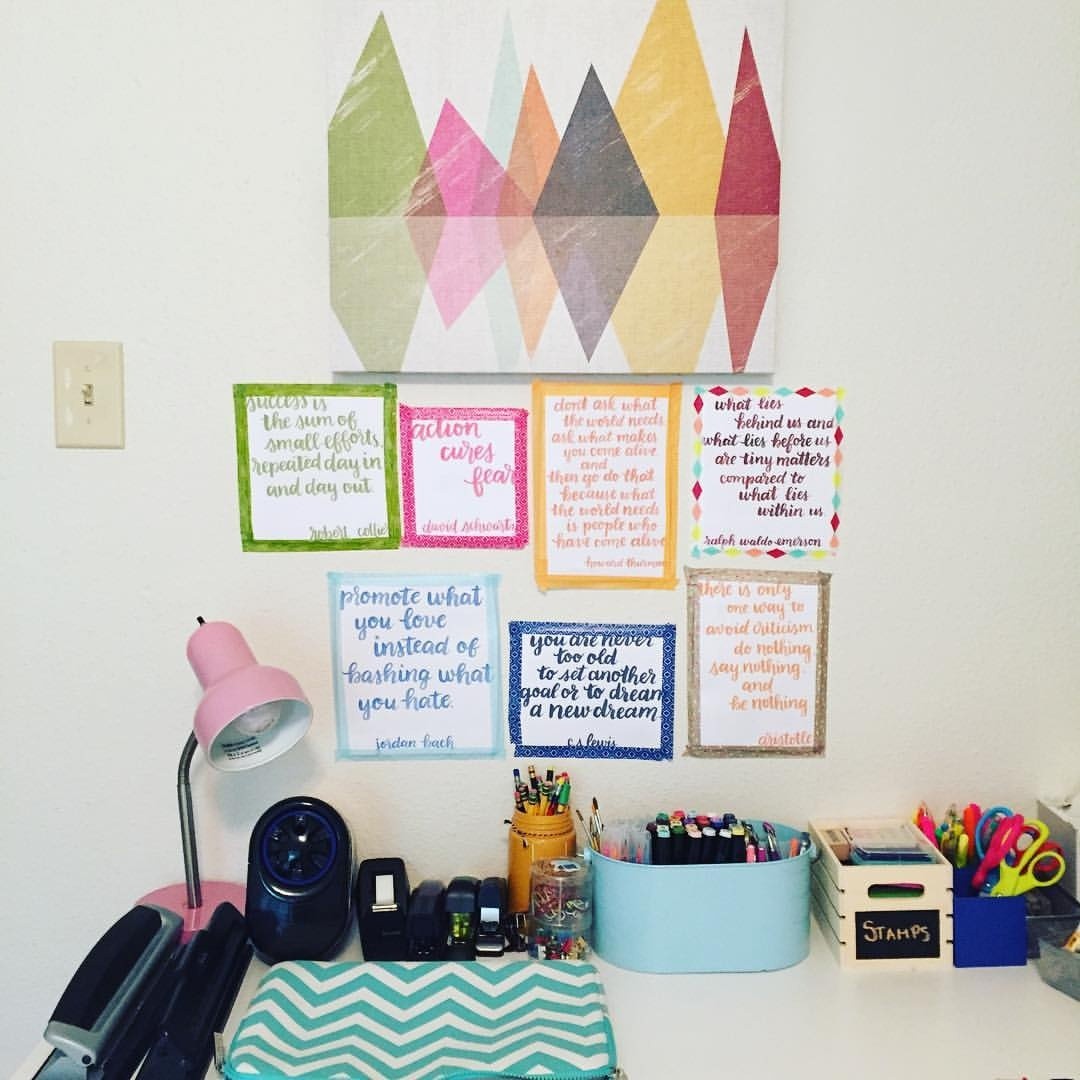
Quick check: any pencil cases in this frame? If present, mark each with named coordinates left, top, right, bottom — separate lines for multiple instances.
left=212, top=957, right=629, bottom=1080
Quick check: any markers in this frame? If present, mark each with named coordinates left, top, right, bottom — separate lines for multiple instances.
left=646, top=809, right=748, bottom=865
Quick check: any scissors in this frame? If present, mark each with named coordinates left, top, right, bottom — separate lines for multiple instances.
left=968, top=805, right=1066, bottom=898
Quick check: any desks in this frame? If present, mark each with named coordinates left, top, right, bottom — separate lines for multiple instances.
left=5, top=878, right=1080, bottom=1080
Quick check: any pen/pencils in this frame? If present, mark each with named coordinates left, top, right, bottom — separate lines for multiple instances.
left=576, top=796, right=651, bottom=865
left=511, top=764, right=572, bottom=816
left=914, top=797, right=984, bottom=872
left=742, top=821, right=811, bottom=864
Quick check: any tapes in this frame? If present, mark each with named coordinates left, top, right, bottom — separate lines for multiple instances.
left=374, top=873, right=396, bottom=907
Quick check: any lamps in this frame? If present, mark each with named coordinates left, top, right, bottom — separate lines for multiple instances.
left=132, top=617, right=312, bottom=944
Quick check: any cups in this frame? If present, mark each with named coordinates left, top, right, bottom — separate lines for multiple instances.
left=507, top=806, right=577, bottom=932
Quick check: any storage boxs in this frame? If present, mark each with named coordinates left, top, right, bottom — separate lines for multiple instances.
left=1018, top=879, right=1080, bottom=960
left=1036, top=929, right=1080, bottom=1000
left=527, top=857, right=594, bottom=928
left=524, top=911, right=596, bottom=962
left=584, top=817, right=819, bottom=973
left=946, top=864, right=1030, bottom=971
left=803, top=819, right=957, bottom=971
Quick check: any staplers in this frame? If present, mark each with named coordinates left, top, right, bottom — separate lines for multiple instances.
left=442, top=874, right=477, bottom=963
left=408, top=879, right=445, bottom=959
left=475, top=875, right=508, bottom=956
